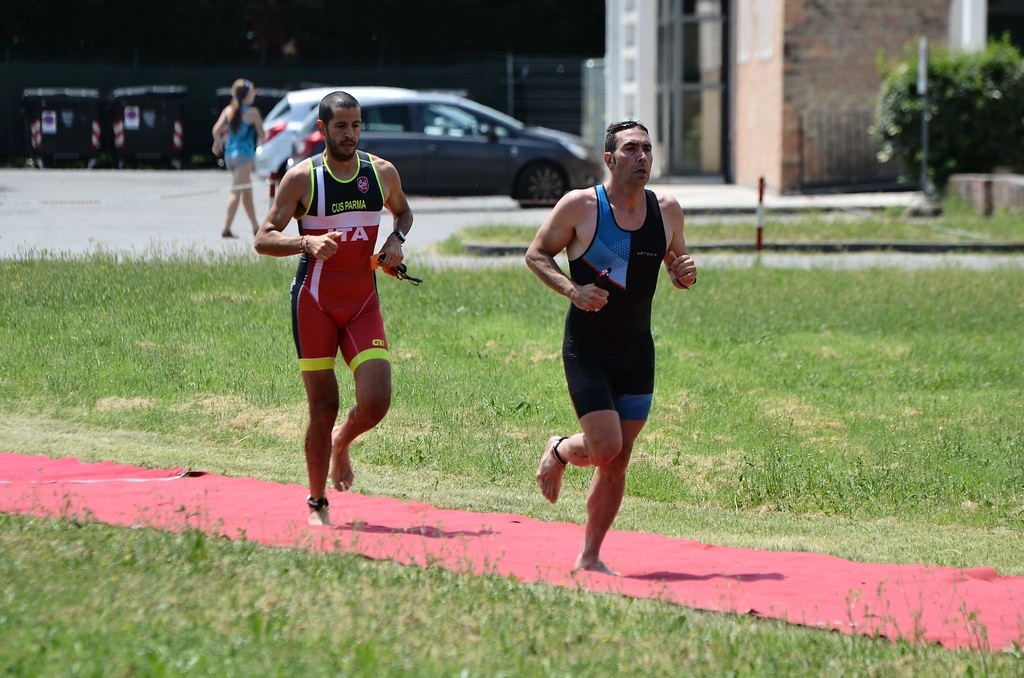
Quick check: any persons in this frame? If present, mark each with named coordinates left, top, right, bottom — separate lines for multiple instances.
left=253, top=92, right=414, bottom=527
left=211, top=78, right=265, bottom=237
left=525, top=121, right=698, bottom=577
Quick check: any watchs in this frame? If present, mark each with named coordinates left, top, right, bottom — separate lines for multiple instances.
left=393, top=231, right=406, bottom=244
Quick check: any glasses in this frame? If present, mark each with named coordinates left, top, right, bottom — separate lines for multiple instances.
left=377, top=255, right=407, bottom=276
left=583, top=268, right=614, bottom=320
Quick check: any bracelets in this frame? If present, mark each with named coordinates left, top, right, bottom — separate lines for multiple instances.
left=676, top=277, right=697, bottom=290
left=304, top=234, right=310, bottom=252
left=300, top=235, right=304, bottom=252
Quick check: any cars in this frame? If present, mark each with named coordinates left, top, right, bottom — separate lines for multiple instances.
left=253, top=85, right=483, bottom=178
left=275, top=98, right=604, bottom=208
